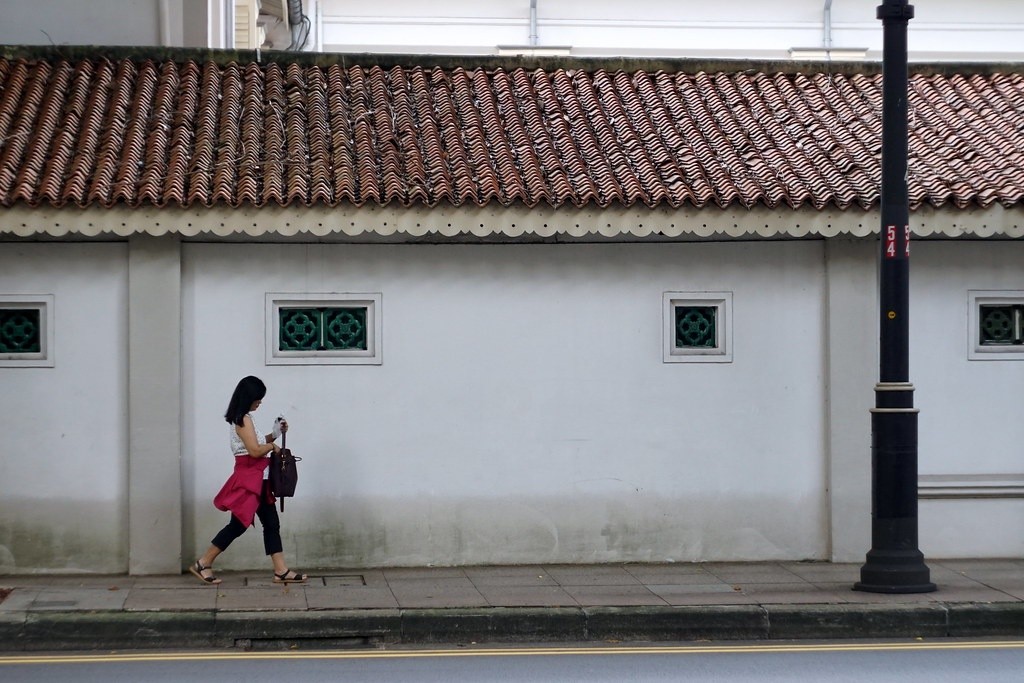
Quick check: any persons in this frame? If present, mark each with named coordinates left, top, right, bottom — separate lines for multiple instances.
left=190, top=376, right=306, bottom=583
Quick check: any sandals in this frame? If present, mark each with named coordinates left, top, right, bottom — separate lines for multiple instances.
left=189, top=561, right=222, bottom=584
left=272, top=569, right=307, bottom=583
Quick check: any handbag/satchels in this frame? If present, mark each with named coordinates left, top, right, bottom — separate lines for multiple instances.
left=269, top=448, right=302, bottom=497
